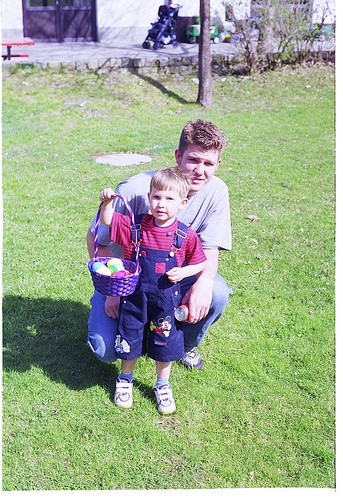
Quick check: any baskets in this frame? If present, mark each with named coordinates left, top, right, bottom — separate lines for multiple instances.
left=88, top=194, right=142, bottom=297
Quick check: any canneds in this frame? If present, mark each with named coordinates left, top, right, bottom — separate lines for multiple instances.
left=174, top=303, right=189, bottom=322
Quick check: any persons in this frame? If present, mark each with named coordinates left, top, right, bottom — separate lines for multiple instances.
left=99, top=168, right=208, bottom=416
left=84, top=119, right=234, bottom=370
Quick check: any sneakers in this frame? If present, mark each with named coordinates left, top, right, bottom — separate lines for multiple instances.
left=182, top=348, right=205, bottom=372
left=154, top=384, right=176, bottom=415
left=114, top=374, right=136, bottom=409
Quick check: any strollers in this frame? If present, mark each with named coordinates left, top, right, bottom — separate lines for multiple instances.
left=142, top=5, right=181, bottom=50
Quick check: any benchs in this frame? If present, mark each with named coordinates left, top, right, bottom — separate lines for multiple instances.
left=2, top=52, right=29, bottom=58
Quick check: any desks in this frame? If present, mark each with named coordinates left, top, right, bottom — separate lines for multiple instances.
left=2, top=37, right=35, bottom=61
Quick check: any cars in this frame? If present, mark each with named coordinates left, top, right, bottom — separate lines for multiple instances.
left=256, top=23, right=335, bottom=64
